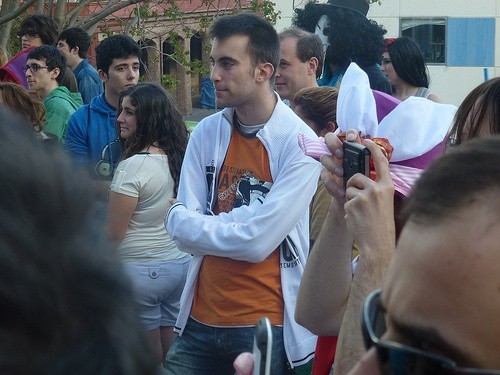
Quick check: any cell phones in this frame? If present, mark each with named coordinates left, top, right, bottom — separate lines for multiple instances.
left=251, top=317, right=272, bottom=375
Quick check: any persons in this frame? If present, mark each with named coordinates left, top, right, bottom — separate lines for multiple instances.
left=106, top=84, right=190, bottom=370
left=16, top=14, right=79, bottom=94
left=164, top=12, right=328, bottom=375
left=56, top=28, right=104, bottom=105
left=273, top=28, right=324, bottom=109
left=0, top=82, right=64, bottom=163
left=295, top=75, right=500, bottom=375
left=379, top=36, right=441, bottom=103
left=294, top=0, right=392, bottom=96
left=348, top=135, right=499, bottom=375
left=24, top=45, right=84, bottom=146
left=291, top=85, right=340, bottom=138
left=65, top=35, right=139, bottom=230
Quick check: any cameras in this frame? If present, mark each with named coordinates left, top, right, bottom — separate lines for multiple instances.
left=343, top=140, right=371, bottom=192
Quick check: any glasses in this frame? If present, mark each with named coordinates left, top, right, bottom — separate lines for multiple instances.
left=444, top=138, right=459, bottom=152
left=17, top=31, right=37, bottom=39
left=357, top=288, right=500, bottom=375
left=24, top=63, right=48, bottom=72
